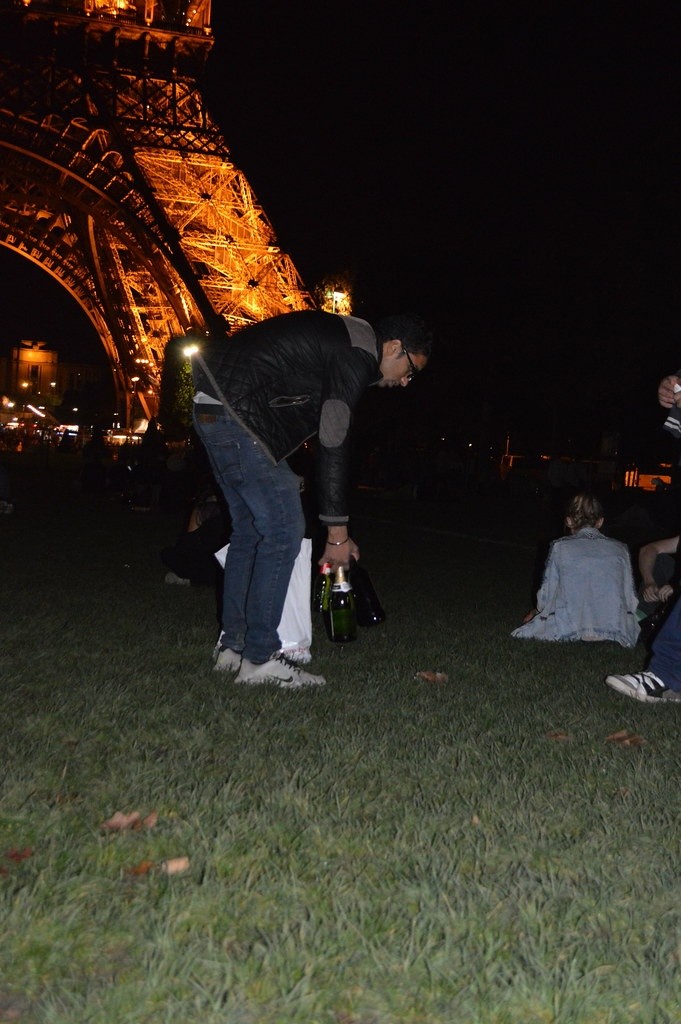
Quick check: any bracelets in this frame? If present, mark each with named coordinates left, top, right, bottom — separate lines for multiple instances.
left=326, top=536, right=350, bottom=545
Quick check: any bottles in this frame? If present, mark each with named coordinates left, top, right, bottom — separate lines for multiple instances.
left=328, top=566, right=359, bottom=643
left=348, top=555, right=385, bottom=627
left=314, top=562, right=330, bottom=612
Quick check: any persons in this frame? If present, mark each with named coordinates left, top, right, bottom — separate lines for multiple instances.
left=190, top=308, right=434, bottom=689
left=521, top=367, right=680, bottom=704
left=164, top=485, right=232, bottom=593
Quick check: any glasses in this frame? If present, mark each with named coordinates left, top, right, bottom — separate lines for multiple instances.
left=403, top=347, right=418, bottom=381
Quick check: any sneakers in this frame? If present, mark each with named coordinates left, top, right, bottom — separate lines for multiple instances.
left=606, top=669, right=681, bottom=702
left=213, top=647, right=242, bottom=673
left=234, top=658, right=326, bottom=692
